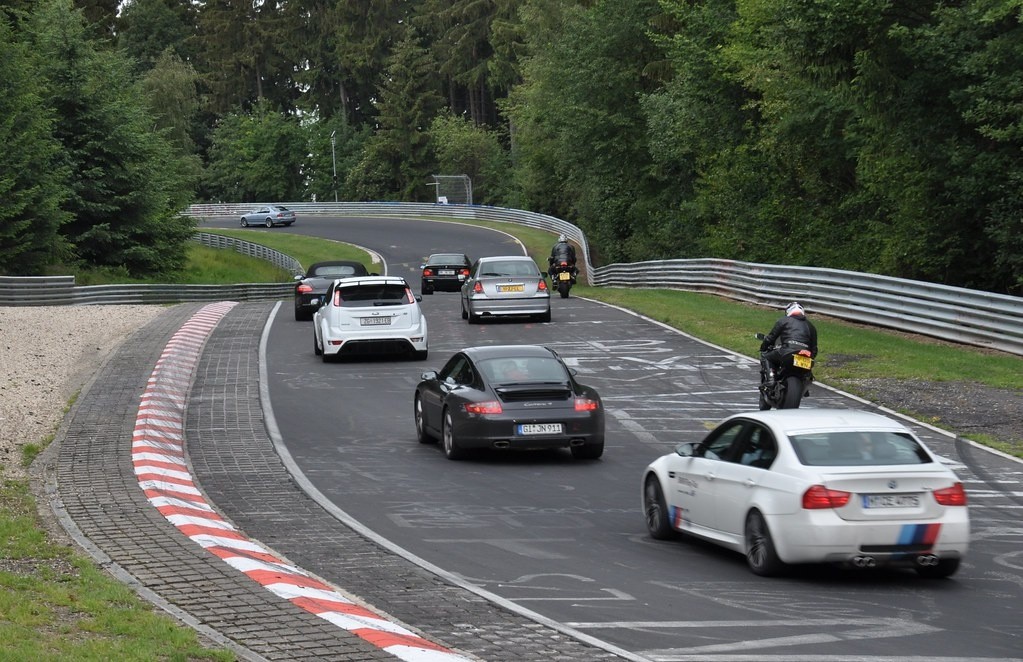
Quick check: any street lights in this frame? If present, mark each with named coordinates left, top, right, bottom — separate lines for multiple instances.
left=331, top=129, right=339, bottom=202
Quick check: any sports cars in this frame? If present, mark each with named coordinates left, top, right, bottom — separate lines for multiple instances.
left=293, top=260, right=380, bottom=322
left=311, top=274, right=430, bottom=363
left=413, top=345, right=607, bottom=460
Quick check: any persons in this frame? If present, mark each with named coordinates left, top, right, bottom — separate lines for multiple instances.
left=547, top=233, right=580, bottom=290
left=741, top=430, right=772, bottom=464
left=759, top=301, right=818, bottom=397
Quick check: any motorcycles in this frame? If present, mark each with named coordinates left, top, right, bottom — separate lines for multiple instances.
left=754, top=333, right=814, bottom=412
left=545, top=257, right=578, bottom=298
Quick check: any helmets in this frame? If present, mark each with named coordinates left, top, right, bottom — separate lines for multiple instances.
left=558, top=235, right=568, bottom=243
left=785, top=301, right=804, bottom=316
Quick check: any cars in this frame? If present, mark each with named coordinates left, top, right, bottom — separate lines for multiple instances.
left=457, top=256, right=553, bottom=325
left=239, top=205, right=296, bottom=228
left=639, top=411, right=970, bottom=578
left=419, top=253, right=473, bottom=295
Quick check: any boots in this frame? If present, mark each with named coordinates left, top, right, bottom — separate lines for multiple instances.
left=552, top=276, right=557, bottom=290
left=758, top=360, right=775, bottom=391
left=571, top=272, right=578, bottom=284
